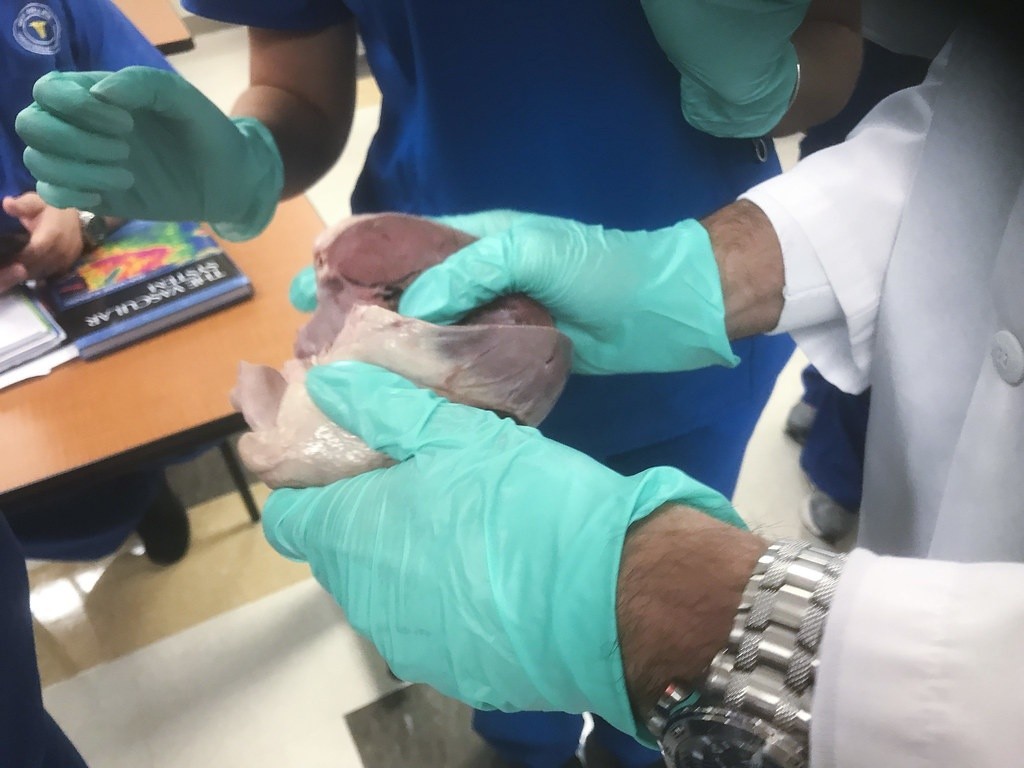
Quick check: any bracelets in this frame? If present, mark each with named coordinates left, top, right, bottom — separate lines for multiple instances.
left=786, top=59, right=801, bottom=120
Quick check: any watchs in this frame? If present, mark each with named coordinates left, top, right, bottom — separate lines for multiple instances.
left=645, top=536, right=848, bottom=768
left=77, top=205, right=109, bottom=253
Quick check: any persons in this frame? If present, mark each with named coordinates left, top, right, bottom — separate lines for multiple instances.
left=0, top=0, right=205, bottom=561
left=0, top=507, right=94, bottom=766
left=776, top=0, right=966, bottom=551
left=14, top=0, right=871, bottom=768
left=255, top=0, right=1024, bottom=767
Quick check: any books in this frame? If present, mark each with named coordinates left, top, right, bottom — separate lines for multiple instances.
left=38, top=218, right=253, bottom=362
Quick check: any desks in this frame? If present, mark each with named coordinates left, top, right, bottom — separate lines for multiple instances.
left=113, top=0, right=194, bottom=55
left=1, top=193, right=331, bottom=523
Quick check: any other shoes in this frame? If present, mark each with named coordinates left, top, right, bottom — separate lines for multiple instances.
left=784, top=397, right=861, bottom=545
left=135, top=483, right=190, bottom=565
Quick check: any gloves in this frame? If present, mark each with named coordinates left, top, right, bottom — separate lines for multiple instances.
left=260, top=359, right=753, bottom=752
left=288, top=208, right=743, bottom=377
left=638, top=1, right=810, bottom=140
left=13, top=63, right=283, bottom=245
